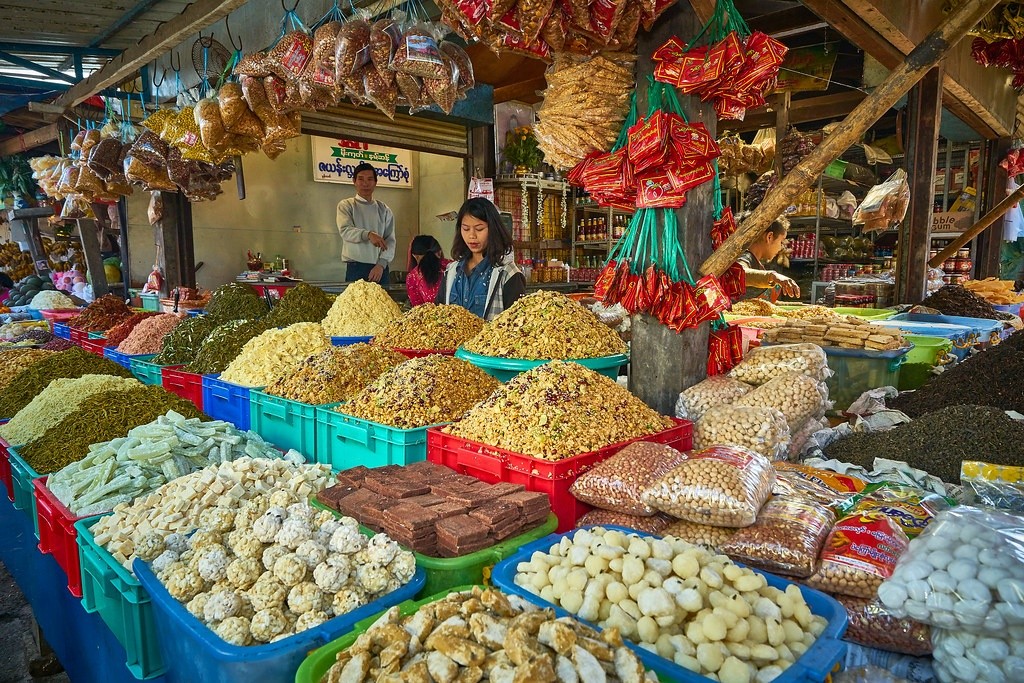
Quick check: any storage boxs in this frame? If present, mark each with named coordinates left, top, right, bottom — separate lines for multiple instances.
left=826, top=159, right=849, bottom=179
left=932, top=211, right=974, bottom=233
left=0, top=293, right=1024, bottom=683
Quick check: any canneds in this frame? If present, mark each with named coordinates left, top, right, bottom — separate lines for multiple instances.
left=788, top=239, right=971, bottom=308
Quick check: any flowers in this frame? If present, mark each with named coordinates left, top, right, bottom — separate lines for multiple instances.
left=496, top=124, right=545, bottom=169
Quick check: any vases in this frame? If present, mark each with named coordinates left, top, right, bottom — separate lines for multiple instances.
left=513, top=165, right=531, bottom=178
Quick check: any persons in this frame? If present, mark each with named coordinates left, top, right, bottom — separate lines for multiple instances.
left=436, top=197, right=526, bottom=319
left=0, top=278, right=14, bottom=304
left=406, top=235, right=453, bottom=306
left=739, top=214, right=801, bottom=302
left=335, top=164, right=396, bottom=285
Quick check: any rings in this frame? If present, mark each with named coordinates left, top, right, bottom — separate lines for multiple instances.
left=786, top=284, right=790, bottom=288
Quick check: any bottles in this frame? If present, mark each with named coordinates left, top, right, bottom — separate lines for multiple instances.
left=824, top=282, right=835, bottom=307
left=273, top=254, right=283, bottom=272
left=516, top=258, right=573, bottom=285
left=573, top=186, right=634, bottom=282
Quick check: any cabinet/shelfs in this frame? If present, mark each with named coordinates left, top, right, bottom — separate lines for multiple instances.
left=494, top=172, right=634, bottom=285
left=784, top=130, right=988, bottom=287
